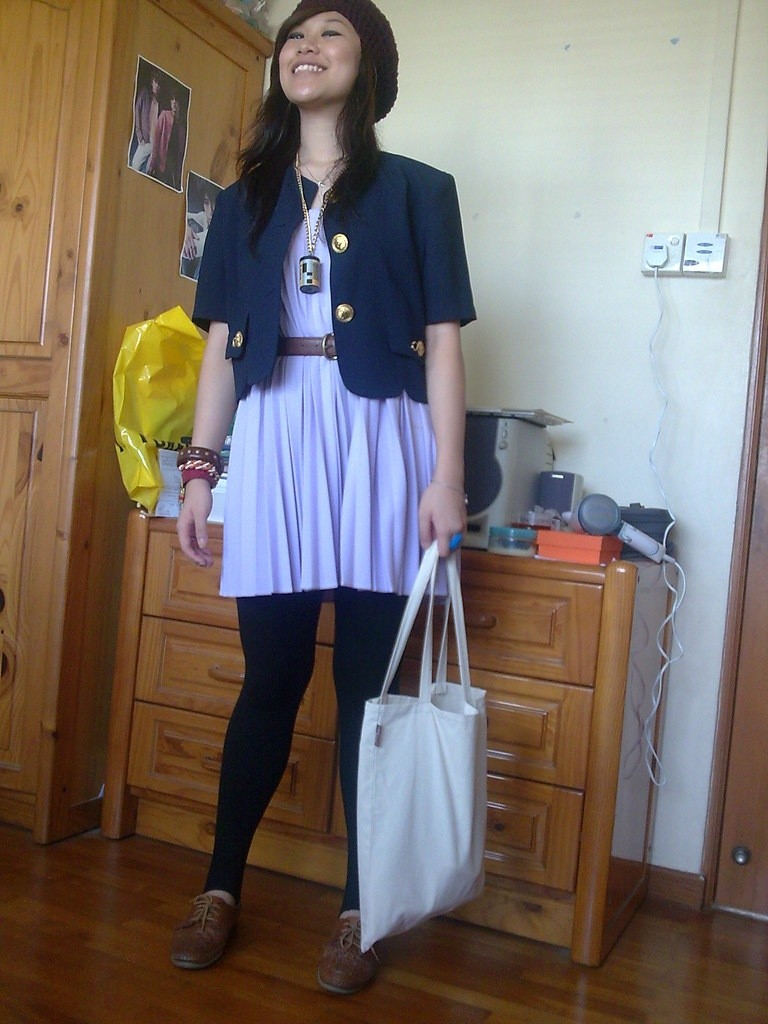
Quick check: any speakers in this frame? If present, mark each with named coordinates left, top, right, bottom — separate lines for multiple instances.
left=462, top=414, right=553, bottom=550
left=537, top=471, right=583, bottom=514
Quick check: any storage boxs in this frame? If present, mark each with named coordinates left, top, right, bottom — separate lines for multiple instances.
left=535, top=529, right=623, bottom=565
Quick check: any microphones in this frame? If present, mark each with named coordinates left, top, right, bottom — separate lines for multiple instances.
left=578, top=494, right=666, bottom=564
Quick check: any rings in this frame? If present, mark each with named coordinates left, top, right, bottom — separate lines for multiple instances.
left=189, top=247, right=192, bottom=249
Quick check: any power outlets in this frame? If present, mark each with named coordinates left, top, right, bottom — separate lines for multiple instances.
left=640, top=232, right=685, bottom=277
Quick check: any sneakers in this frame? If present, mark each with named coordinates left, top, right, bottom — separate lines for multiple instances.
left=317, top=915, right=385, bottom=993
left=170, top=892, right=240, bottom=970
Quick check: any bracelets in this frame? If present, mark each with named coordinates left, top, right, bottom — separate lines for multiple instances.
left=431, top=481, right=469, bottom=504
left=176, top=447, right=224, bottom=502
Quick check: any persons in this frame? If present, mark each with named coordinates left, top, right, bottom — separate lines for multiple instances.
left=182, top=185, right=219, bottom=278
left=170, top=0, right=475, bottom=995
left=132, top=74, right=185, bottom=186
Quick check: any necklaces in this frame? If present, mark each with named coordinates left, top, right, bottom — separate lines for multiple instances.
left=302, top=161, right=330, bottom=187
left=294, top=152, right=332, bottom=293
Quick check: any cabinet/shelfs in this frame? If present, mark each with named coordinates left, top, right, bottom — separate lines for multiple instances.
left=0, top=0, right=276, bottom=843
left=102, top=509, right=679, bottom=970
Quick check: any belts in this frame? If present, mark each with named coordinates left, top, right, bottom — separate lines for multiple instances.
left=276, top=334, right=337, bottom=360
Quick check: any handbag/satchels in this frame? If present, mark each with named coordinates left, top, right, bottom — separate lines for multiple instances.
left=112, top=306, right=207, bottom=514
left=356, top=538, right=487, bottom=954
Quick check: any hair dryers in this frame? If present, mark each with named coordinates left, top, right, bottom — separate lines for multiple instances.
left=577, top=493, right=675, bottom=566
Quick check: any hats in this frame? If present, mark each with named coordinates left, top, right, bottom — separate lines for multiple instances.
left=283, top=0, right=399, bottom=123
